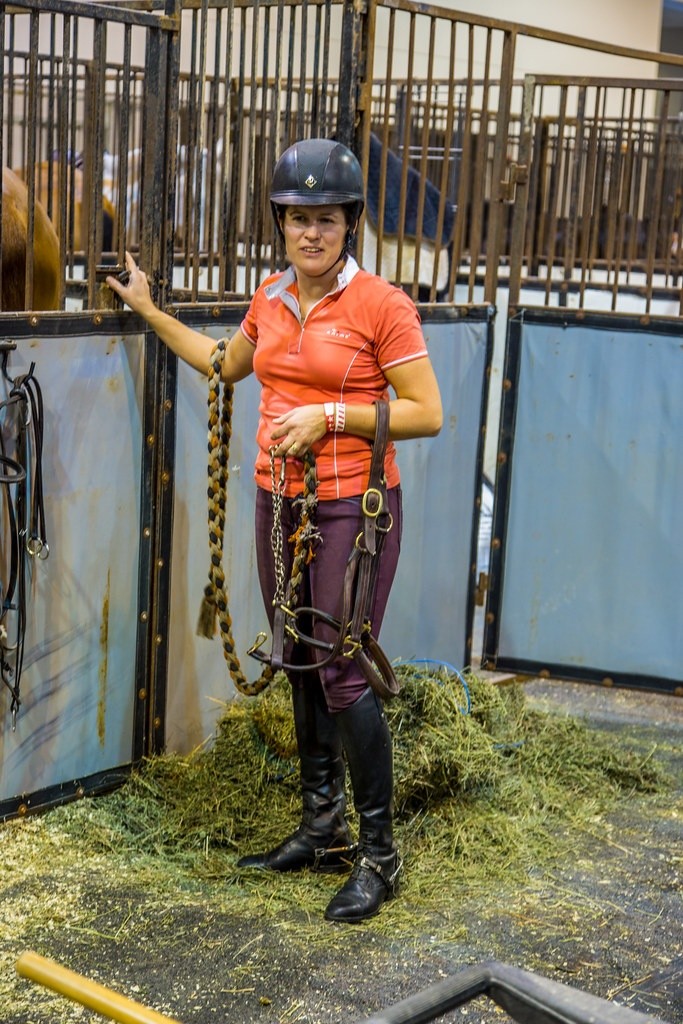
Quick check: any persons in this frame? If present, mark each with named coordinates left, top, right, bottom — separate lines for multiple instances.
left=106, top=137, right=443, bottom=923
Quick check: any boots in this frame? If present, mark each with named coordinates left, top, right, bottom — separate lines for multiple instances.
left=326, top=683, right=398, bottom=921
left=235, top=681, right=355, bottom=876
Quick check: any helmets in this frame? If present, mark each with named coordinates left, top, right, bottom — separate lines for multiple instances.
left=269, top=138, right=364, bottom=220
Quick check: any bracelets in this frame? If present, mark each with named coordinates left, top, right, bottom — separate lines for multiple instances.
left=323, top=402, right=345, bottom=432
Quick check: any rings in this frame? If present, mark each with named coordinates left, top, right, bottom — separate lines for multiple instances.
left=291, top=445, right=299, bottom=452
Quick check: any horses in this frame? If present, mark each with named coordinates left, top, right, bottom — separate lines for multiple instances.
left=324, top=125, right=667, bottom=263
left=0, top=159, right=132, bottom=313
